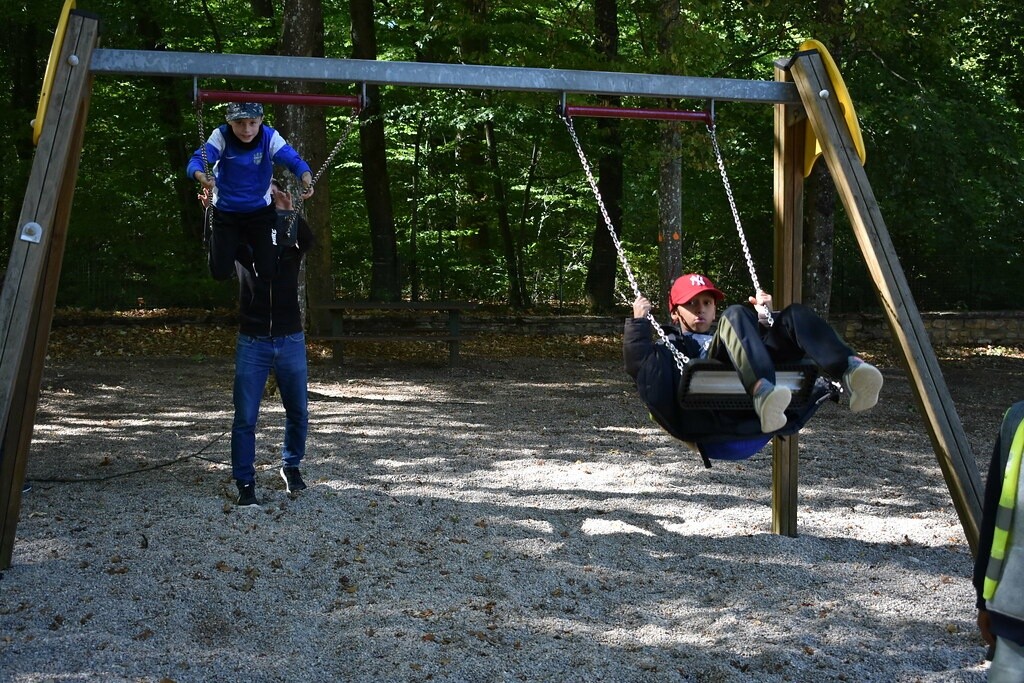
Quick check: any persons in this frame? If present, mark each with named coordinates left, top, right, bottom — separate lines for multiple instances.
left=623, top=273, right=884, bottom=460
left=186, top=91, right=315, bottom=282
left=971, top=400, right=1024, bottom=683
left=197, top=181, right=308, bottom=505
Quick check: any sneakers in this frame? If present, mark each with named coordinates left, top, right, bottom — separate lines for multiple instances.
left=279, top=466, right=307, bottom=494
left=840, top=357, right=882, bottom=412
left=754, top=378, right=791, bottom=433
left=236, top=480, right=259, bottom=508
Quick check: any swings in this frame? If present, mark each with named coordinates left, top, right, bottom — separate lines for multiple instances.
left=563, top=119, right=883, bottom=467
left=198, top=103, right=361, bottom=252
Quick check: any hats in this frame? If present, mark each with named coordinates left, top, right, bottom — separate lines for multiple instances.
left=668, top=274, right=724, bottom=312
left=226, top=101, right=264, bottom=120
